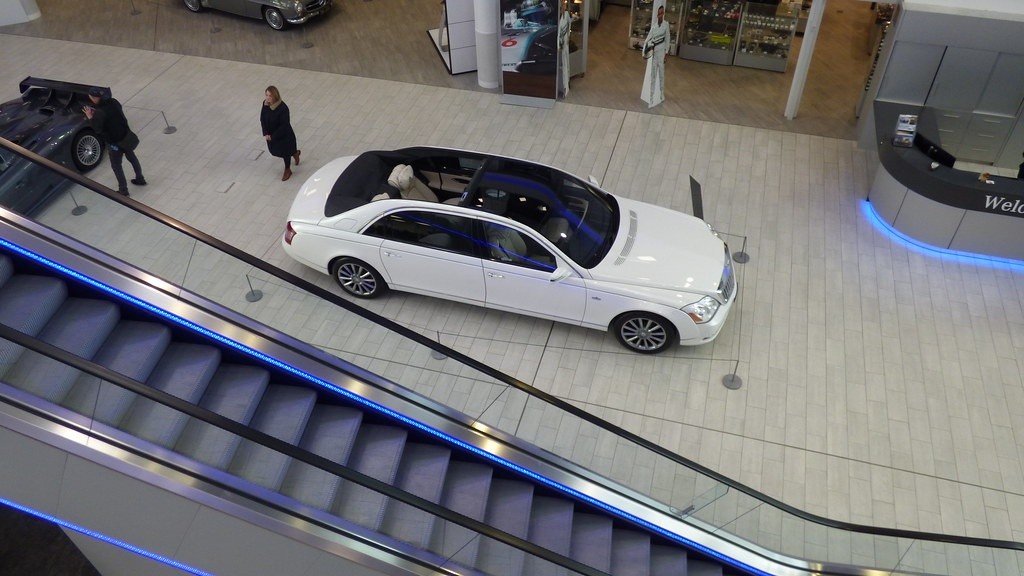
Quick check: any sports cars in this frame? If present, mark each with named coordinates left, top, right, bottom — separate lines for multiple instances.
left=0, top=76, right=113, bottom=219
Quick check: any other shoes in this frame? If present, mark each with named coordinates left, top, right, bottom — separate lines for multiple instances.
left=131, top=179, right=146, bottom=185
left=282, top=170, right=292, bottom=181
left=293, top=150, right=300, bottom=165
left=116, top=191, right=129, bottom=196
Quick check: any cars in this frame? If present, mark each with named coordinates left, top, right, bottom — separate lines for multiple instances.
left=184, top=0, right=333, bottom=30
left=283, top=145, right=737, bottom=354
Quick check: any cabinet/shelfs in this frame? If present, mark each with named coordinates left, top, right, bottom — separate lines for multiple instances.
left=627, top=0, right=801, bottom=74
left=856, top=21, right=891, bottom=117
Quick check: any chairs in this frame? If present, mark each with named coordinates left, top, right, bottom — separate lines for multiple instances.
left=370, top=164, right=462, bottom=224
left=484, top=221, right=556, bottom=268
left=370, top=192, right=450, bottom=248
left=537, top=216, right=572, bottom=247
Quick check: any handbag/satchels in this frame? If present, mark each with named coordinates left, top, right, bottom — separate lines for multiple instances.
left=118, top=130, right=140, bottom=153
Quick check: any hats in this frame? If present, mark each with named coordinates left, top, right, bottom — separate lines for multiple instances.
left=88, top=87, right=109, bottom=100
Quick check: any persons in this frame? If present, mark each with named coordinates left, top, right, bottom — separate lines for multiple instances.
left=82, top=88, right=147, bottom=197
left=559, top=0, right=571, bottom=96
left=260, top=85, right=301, bottom=181
left=642, top=5, right=670, bottom=106
left=698, top=0, right=723, bottom=32
